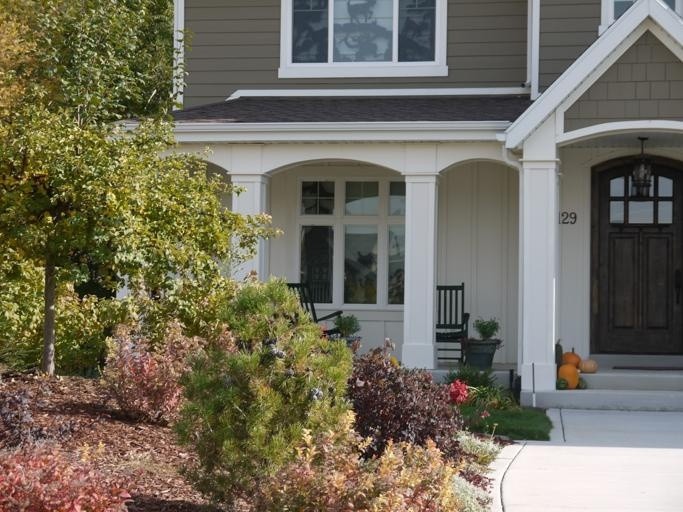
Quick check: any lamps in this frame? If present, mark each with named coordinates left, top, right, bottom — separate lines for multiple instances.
left=631, top=136, right=651, bottom=186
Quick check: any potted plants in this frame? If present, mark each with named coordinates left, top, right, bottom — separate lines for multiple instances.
left=464, top=316, right=502, bottom=370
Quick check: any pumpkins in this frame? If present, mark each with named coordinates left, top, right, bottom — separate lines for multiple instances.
left=558, top=364, right=579, bottom=389
left=563, top=348, right=580, bottom=367
left=579, top=361, right=598, bottom=372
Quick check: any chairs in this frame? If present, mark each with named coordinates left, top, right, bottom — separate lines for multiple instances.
left=436, top=282, right=470, bottom=365
left=282, top=282, right=344, bottom=340
